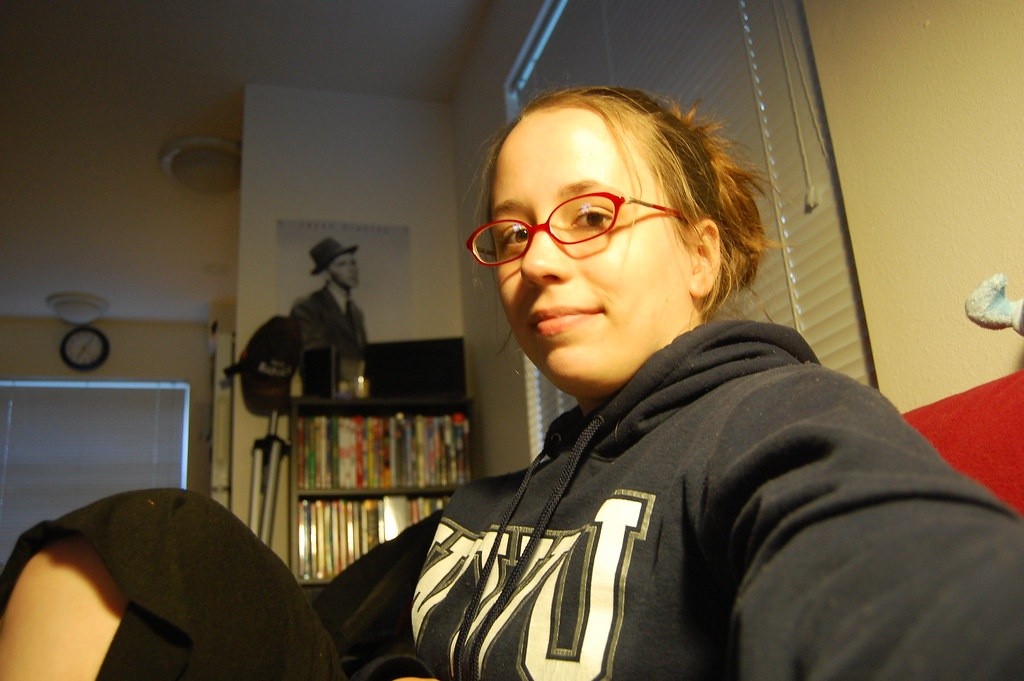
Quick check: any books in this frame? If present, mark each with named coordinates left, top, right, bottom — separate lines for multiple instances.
left=298, top=413, right=471, bottom=580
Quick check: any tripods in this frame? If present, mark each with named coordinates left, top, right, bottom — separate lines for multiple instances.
left=248, top=397, right=292, bottom=542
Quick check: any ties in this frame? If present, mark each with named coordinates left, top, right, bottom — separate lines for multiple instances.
left=345, top=300, right=355, bottom=328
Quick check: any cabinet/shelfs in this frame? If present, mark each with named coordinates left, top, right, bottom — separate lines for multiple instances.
left=289, top=393, right=486, bottom=591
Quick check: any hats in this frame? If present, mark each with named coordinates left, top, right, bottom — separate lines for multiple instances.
left=310, top=237, right=357, bottom=276
left=225, top=317, right=301, bottom=415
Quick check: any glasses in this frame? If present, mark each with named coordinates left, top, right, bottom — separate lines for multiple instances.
left=465, top=191, right=688, bottom=266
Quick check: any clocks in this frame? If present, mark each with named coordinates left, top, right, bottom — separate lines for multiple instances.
left=60, top=325, right=111, bottom=371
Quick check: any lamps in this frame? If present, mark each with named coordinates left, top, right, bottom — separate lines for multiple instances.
left=166, top=136, right=243, bottom=200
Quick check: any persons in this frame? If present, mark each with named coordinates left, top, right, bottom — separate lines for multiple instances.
left=291, top=236, right=368, bottom=349
left=0, top=82, right=1024, bottom=681
